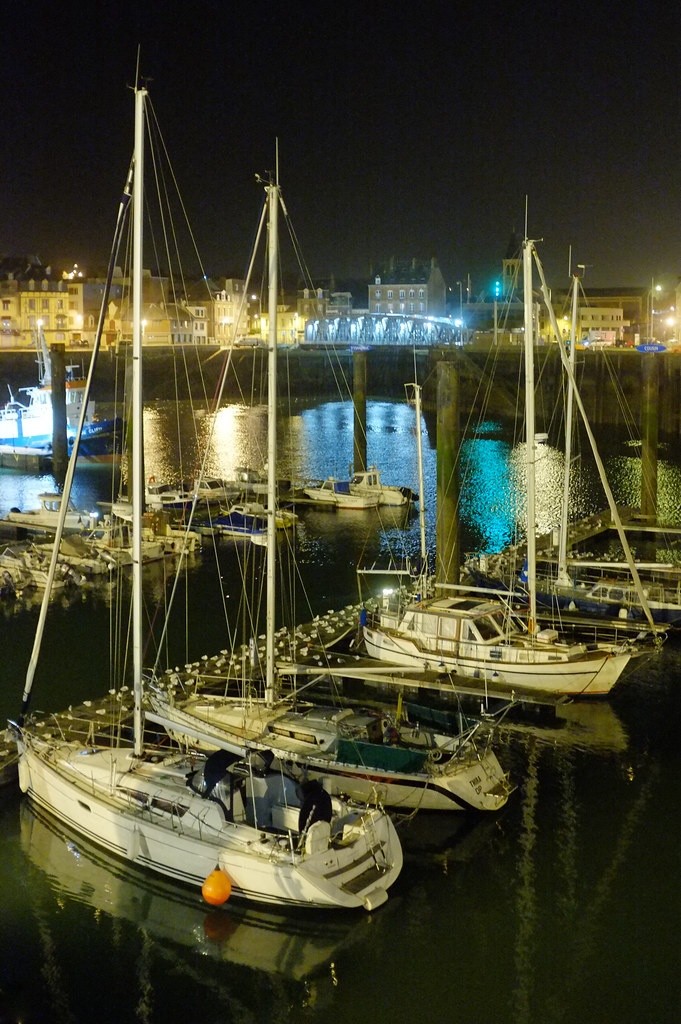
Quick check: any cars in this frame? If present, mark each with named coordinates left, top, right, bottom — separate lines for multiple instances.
left=566, top=338, right=635, bottom=351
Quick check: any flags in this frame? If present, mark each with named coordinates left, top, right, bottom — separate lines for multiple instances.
left=518, top=559, right=528, bottom=583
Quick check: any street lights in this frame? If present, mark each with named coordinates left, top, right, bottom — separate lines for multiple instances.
left=252, top=295, right=262, bottom=320
left=647, top=286, right=662, bottom=345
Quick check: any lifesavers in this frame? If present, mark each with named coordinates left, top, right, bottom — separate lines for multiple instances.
left=359, top=608, right=369, bottom=627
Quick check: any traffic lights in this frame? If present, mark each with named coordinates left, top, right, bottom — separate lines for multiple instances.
left=496, top=282, right=500, bottom=297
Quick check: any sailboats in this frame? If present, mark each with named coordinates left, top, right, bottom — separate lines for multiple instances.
left=364, top=659, right=627, bottom=881
left=360, top=193, right=680, bottom=696
left=20, top=801, right=372, bottom=983
left=145, top=137, right=522, bottom=814
left=7, top=38, right=405, bottom=913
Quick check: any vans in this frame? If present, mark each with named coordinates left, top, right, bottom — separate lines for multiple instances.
left=233, top=337, right=258, bottom=348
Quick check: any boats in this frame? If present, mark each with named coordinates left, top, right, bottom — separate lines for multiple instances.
left=1, top=465, right=413, bottom=589
left=0, top=331, right=129, bottom=457
left=9, top=463, right=290, bottom=530
